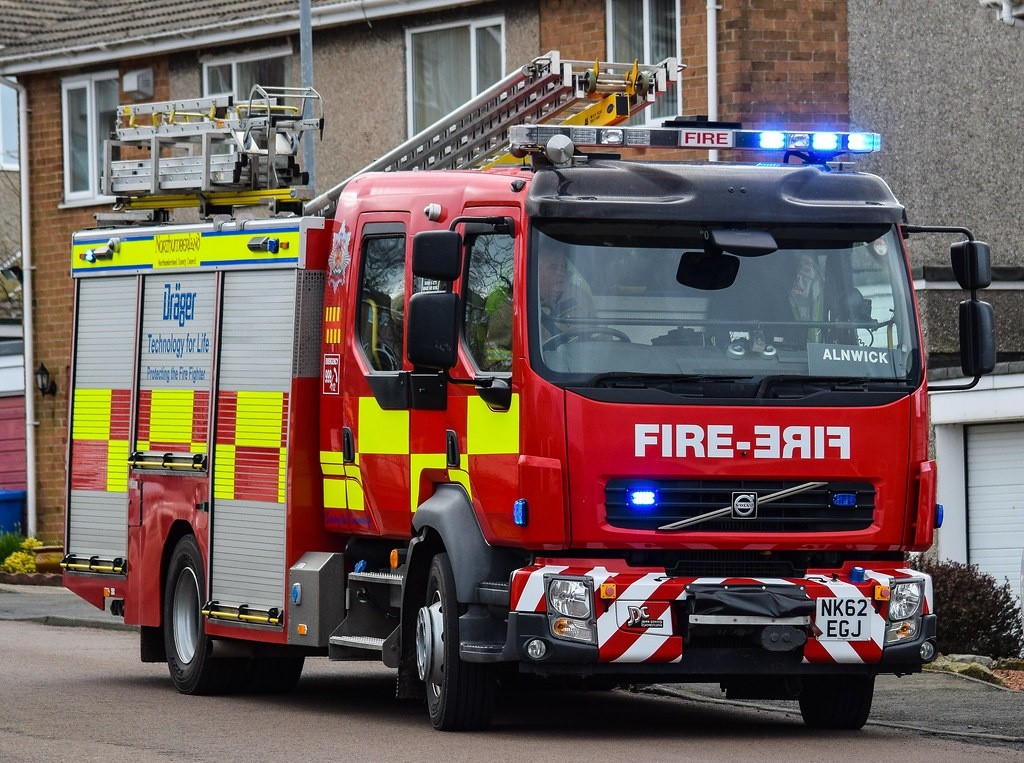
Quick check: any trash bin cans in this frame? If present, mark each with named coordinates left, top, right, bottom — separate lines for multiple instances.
left=0, top=490, right=26, bottom=539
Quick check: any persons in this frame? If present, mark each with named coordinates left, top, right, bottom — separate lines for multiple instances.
left=483, top=244, right=612, bottom=371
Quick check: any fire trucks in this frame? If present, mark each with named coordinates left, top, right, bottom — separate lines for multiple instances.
left=57, top=46, right=999, bottom=731
